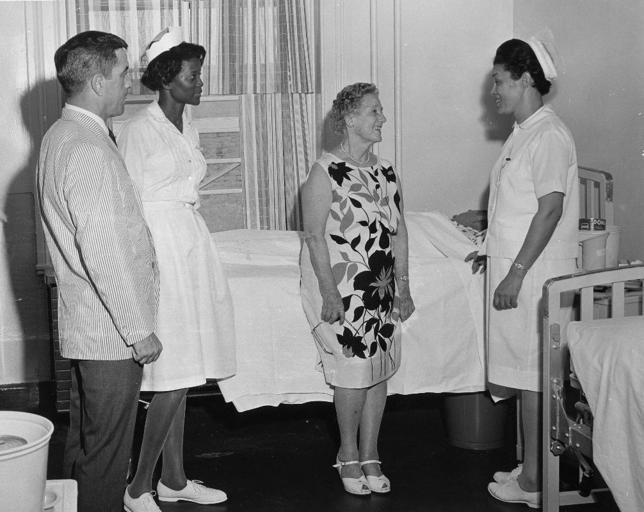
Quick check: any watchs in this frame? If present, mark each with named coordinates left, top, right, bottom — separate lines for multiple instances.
left=394, top=275, right=410, bottom=282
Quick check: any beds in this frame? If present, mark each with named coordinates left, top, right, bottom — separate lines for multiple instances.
left=536, top=265, right=644, bottom=512
left=210, top=168, right=620, bottom=405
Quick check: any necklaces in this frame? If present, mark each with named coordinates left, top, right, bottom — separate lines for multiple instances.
left=339, top=142, right=371, bottom=165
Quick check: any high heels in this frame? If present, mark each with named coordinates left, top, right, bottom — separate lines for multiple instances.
left=332, top=456, right=391, bottom=495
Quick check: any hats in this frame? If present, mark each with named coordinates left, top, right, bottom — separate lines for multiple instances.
left=527, top=27, right=564, bottom=82
left=144, top=25, right=184, bottom=65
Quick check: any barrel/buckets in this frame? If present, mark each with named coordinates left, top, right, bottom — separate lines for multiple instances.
left=0, top=410, right=54, bottom=512
left=444, top=393, right=507, bottom=449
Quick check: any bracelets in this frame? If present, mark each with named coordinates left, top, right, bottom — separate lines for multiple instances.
left=513, top=263, right=528, bottom=270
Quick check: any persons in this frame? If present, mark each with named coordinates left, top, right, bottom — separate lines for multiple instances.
left=464, top=40, right=581, bottom=509
left=33, top=30, right=164, bottom=510
left=449, top=209, right=488, bottom=248
left=118, top=42, right=236, bottom=511
left=299, top=83, right=414, bottom=495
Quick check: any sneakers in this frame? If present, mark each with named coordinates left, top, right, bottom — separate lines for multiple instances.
left=123, top=477, right=228, bottom=512
left=487, top=463, right=542, bottom=509
